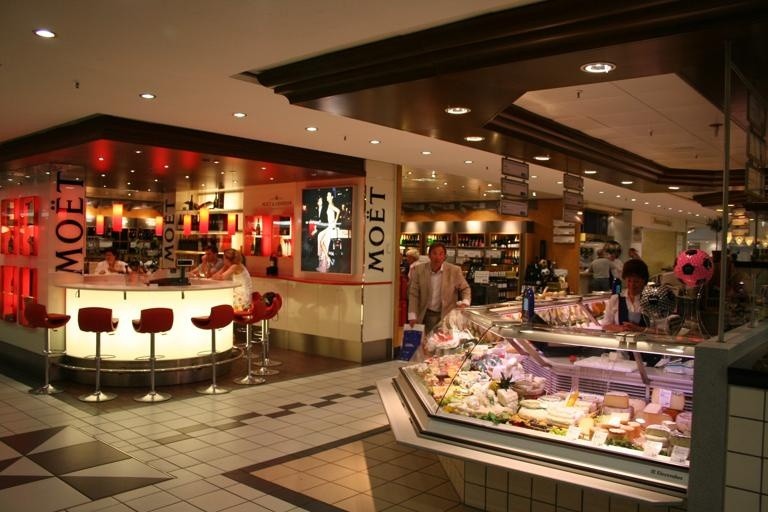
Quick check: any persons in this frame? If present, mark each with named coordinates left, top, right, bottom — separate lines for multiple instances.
left=404, top=247, right=431, bottom=280
left=407, top=240, right=471, bottom=336
left=606, top=249, right=625, bottom=292
left=307, top=195, right=335, bottom=270
left=212, top=248, right=253, bottom=313
left=95, top=246, right=132, bottom=275
left=628, top=248, right=640, bottom=260
left=306, top=190, right=341, bottom=273
left=603, top=256, right=670, bottom=368
left=586, top=248, right=619, bottom=293
left=188, top=243, right=224, bottom=279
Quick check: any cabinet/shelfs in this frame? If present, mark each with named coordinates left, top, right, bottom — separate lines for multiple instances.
left=85, top=235, right=163, bottom=264
left=173, top=209, right=242, bottom=256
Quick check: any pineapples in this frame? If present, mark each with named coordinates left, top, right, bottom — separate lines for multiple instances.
left=488, top=371, right=516, bottom=394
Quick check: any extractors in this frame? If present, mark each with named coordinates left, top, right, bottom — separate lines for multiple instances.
left=579, top=233, right=615, bottom=244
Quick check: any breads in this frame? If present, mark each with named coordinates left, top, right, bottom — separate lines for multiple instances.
left=609, top=421, right=642, bottom=444
left=645, top=423, right=692, bottom=461
left=604, top=391, right=630, bottom=409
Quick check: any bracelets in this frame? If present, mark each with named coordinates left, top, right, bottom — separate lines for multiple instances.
left=643, top=327, right=649, bottom=334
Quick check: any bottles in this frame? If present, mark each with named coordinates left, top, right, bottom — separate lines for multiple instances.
left=191, top=215, right=199, bottom=230
left=256, top=217, right=260, bottom=235
left=501, top=250, right=518, bottom=264
left=177, top=214, right=185, bottom=230
left=522, top=288, right=534, bottom=322
left=121, top=249, right=130, bottom=262
left=129, top=230, right=153, bottom=240
left=470, top=258, right=483, bottom=272
left=491, top=235, right=519, bottom=248
left=425, top=235, right=452, bottom=245
left=214, top=192, right=218, bottom=208
left=459, top=235, right=484, bottom=246
left=276, top=243, right=283, bottom=256
left=8, top=232, right=15, bottom=253
left=4, top=296, right=16, bottom=322
left=10, top=270, right=14, bottom=295
left=178, top=234, right=198, bottom=250
left=107, top=222, right=112, bottom=237
left=489, top=266, right=518, bottom=302
left=27, top=201, right=34, bottom=225
left=7, top=202, right=14, bottom=225
left=400, top=234, right=420, bottom=245
left=189, top=195, right=193, bottom=210
left=28, top=233, right=35, bottom=256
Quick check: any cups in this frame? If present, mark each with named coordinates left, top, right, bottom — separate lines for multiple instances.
left=193, top=270, right=210, bottom=280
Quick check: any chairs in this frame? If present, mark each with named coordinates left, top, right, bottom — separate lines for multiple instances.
left=24, top=291, right=282, bottom=405
